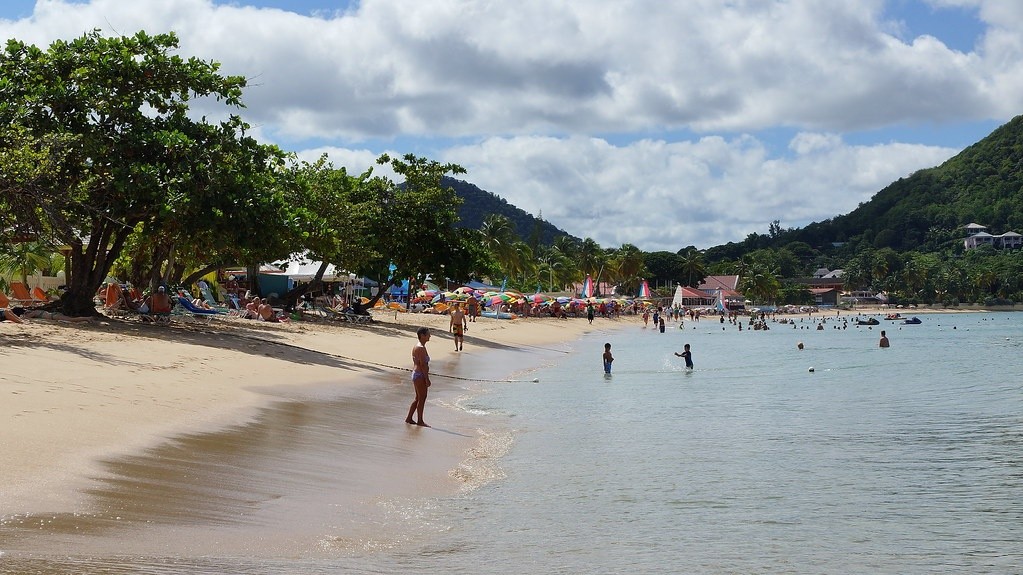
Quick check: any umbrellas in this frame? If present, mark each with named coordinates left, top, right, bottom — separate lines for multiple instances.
left=353, top=284, right=368, bottom=289
left=412, top=287, right=652, bottom=322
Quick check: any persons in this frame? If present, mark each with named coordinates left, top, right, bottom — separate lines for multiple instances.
left=674, top=344, right=693, bottom=369
left=335, top=295, right=369, bottom=315
left=501, top=297, right=701, bottom=333
left=602, top=343, right=614, bottom=375
left=714, top=309, right=890, bottom=349
left=222, top=274, right=290, bottom=323
left=98, top=285, right=211, bottom=322
left=0, top=306, right=95, bottom=324
left=404, top=325, right=431, bottom=428
left=449, top=303, right=467, bottom=351
left=466, top=293, right=477, bottom=322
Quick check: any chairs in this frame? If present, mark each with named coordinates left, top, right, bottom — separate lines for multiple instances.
left=115, top=285, right=373, bottom=327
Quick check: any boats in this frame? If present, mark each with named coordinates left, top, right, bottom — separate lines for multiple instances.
left=883, top=312, right=907, bottom=320
left=902, top=316, right=922, bottom=324
left=856, top=318, right=879, bottom=325
